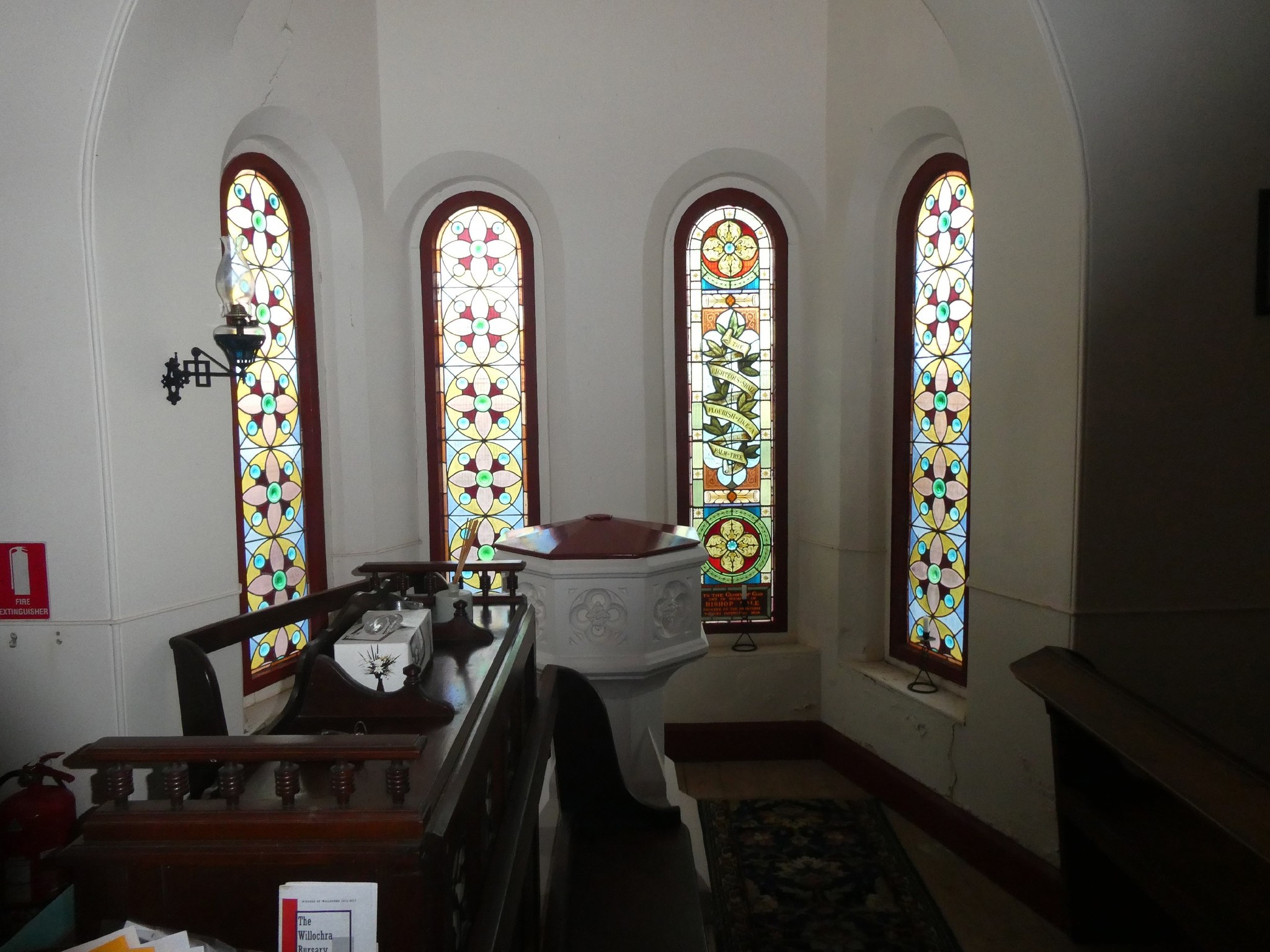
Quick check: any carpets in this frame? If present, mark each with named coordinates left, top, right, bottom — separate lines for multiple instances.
left=698, top=797, right=962, bottom=952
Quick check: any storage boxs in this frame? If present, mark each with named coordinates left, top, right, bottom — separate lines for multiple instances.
left=334, top=609, right=433, bottom=692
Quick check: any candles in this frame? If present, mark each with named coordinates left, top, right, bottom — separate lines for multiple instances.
left=923, top=616, right=930, bottom=632
left=742, top=585, right=747, bottom=599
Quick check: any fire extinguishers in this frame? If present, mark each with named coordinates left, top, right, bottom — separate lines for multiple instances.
left=0, top=751, right=77, bottom=946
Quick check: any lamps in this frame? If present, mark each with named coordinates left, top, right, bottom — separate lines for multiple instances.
left=161, top=236, right=265, bottom=405
